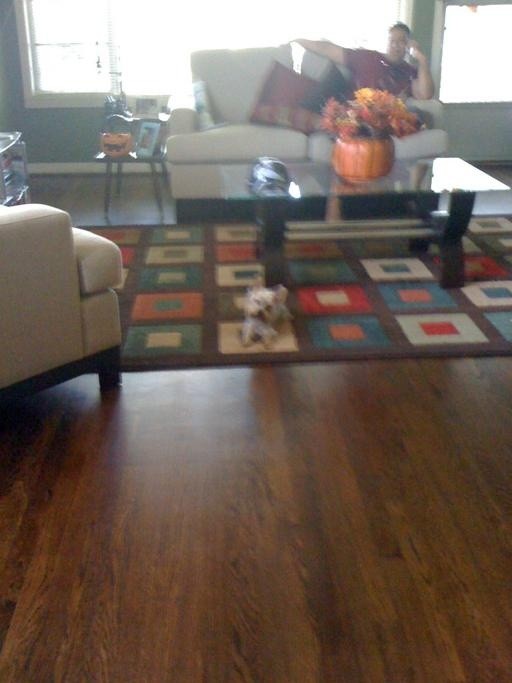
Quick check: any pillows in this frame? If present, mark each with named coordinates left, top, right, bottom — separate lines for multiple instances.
left=245, top=57, right=348, bottom=132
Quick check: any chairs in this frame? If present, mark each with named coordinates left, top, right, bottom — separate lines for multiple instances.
left=0, top=202, right=124, bottom=405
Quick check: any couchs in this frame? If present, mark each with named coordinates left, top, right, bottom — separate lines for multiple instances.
left=159, top=40, right=450, bottom=224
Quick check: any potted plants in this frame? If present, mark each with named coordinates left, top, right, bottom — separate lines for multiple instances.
left=320, top=86, right=423, bottom=184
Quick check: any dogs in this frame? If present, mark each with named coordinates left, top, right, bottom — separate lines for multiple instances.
left=239, top=275, right=294, bottom=351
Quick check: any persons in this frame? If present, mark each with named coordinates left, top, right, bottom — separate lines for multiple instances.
left=288, top=21, right=434, bottom=101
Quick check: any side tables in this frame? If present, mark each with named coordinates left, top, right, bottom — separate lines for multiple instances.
left=97, top=148, right=169, bottom=214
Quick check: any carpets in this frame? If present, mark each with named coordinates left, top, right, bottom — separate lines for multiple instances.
left=72, top=212, right=511, bottom=372
